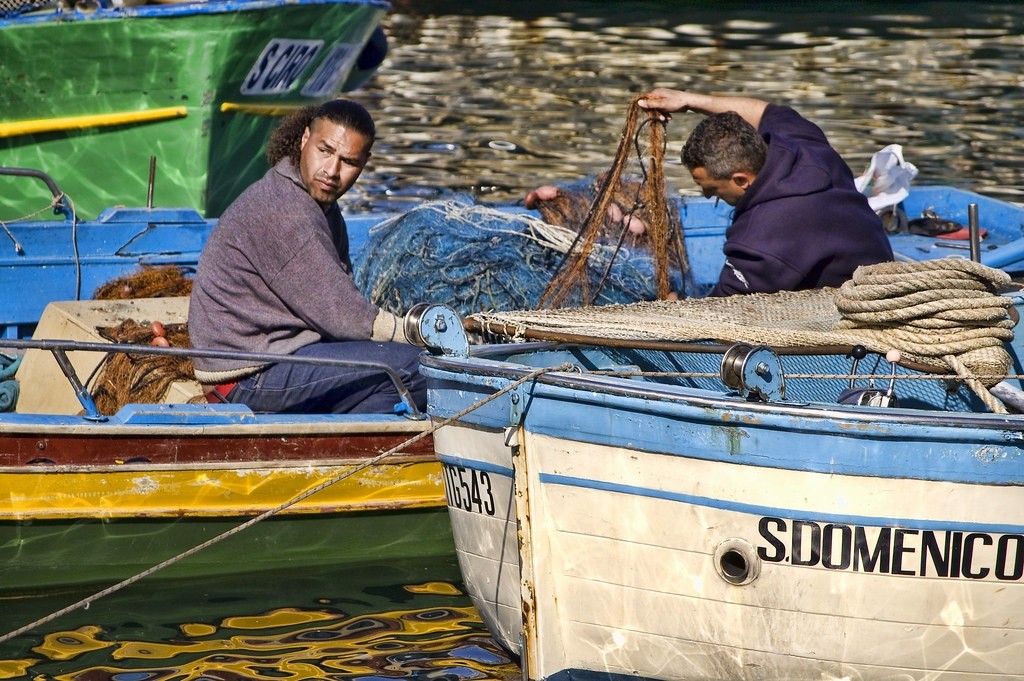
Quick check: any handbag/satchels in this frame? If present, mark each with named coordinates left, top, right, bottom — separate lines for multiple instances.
left=854, top=144, right=919, bottom=215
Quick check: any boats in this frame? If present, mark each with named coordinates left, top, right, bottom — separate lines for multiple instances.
left=400, top=204, right=1024, bottom=681
left=1, top=163, right=1024, bottom=595
left=0, top=0, right=393, bottom=226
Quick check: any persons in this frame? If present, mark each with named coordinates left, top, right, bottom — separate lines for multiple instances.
left=188, top=100, right=428, bottom=417
left=639, top=88, right=894, bottom=302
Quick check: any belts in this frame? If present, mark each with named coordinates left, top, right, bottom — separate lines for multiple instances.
left=203, top=381, right=237, bottom=404
